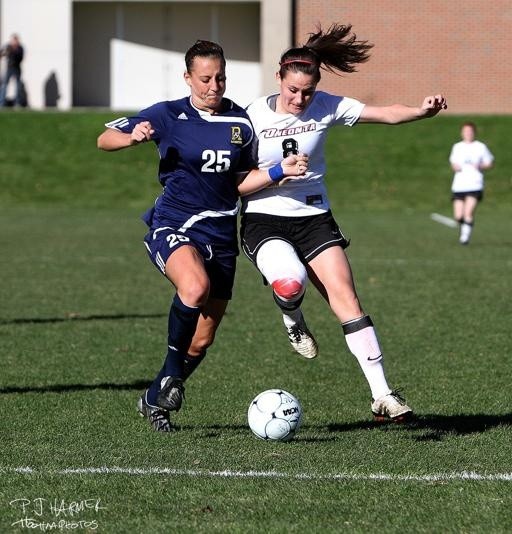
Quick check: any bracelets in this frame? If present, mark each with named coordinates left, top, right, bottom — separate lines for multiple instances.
left=267, top=161, right=287, bottom=184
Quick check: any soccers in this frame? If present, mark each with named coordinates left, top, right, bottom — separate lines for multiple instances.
left=247, top=388, right=303, bottom=442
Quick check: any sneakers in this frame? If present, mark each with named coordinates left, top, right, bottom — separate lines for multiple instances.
left=157, top=376, right=182, bottom=410
left=459, top=237, right=469, bottom=242
left=135, top=389, right=178, bottom=432
left=283, top=310, right=317, bottom=359
left=371, top=392, right=412, bottom=420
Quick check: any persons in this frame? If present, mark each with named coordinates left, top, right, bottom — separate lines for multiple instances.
left=448, top=121, right=496, bottom=244
left=97, top=38, right=310, bottom=435
left=0, top=34, right=24, bottom=108
left=243, top=21, right=446, bottom=421
